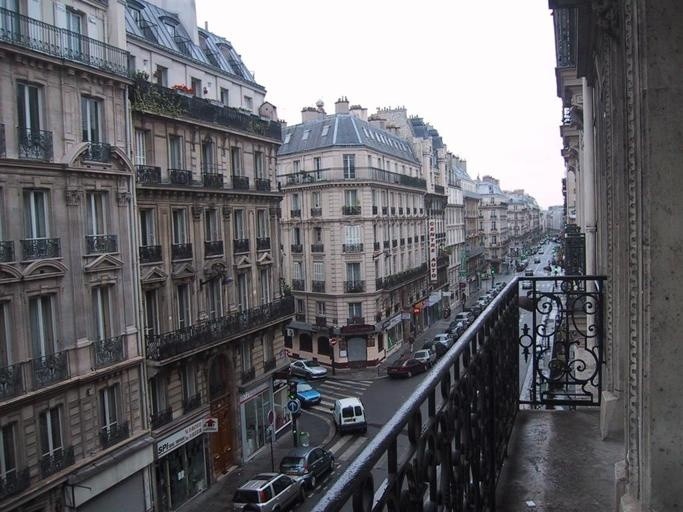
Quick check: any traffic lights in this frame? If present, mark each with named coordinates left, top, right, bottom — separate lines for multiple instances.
left=288, top=385, right=298, bottom=400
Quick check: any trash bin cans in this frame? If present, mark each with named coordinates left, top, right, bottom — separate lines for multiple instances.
left=300, top=432, right=310, bottom=447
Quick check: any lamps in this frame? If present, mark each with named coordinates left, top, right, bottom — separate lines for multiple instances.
left=517, top=288, right=560, bottom=317
left=199, top=263, right=235, bottom=293
left=371, top=249, right=395, bottom=262
left=542, top=260, right=568, bottom=276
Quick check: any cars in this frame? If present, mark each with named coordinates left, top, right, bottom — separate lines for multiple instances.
left=289, top=359, right=327, bottom=382
left=514, top=235, right=550, bottom=289
left=387, top=282, right=507, bottom=378
left=279, top=446, right=336, bottom=488
left=287, top=379, right=321, bottom=408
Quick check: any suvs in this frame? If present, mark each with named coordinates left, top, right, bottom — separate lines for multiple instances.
left=234, top=473, right=306, bottom=511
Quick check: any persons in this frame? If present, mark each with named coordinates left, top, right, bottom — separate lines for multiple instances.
left=408, top=333, right=414, bottom=352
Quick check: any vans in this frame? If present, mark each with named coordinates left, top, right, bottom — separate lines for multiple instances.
left=330, top=397, right=366, bottom=437
